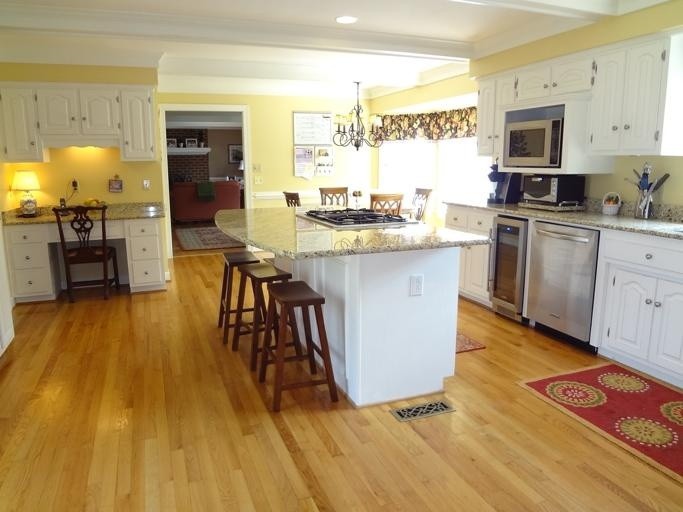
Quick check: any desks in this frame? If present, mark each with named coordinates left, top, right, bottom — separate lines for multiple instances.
left=0, top=200, right=174, bottom=301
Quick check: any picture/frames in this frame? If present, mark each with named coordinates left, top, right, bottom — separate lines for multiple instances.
left=291, top=110, right=333, bottom=147
left=109, top=180, right=123, bottom=193
left=227, top=142, right=242, bottom=165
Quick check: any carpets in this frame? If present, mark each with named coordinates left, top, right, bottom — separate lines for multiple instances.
left=455, top=329, right=484, bottom=355
left=512, top=357, right=682, bottom=485
left=173, top=225, right=248, bottom=252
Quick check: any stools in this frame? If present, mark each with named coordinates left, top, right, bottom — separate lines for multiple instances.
left=229, top=261, right=302, bottom=383
left=256, top=278, right=340, bottom=412
left=214, top=249, right=260, bottom=348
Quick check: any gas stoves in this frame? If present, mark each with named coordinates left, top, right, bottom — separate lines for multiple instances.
left=294, top=208, right=418, bottom=231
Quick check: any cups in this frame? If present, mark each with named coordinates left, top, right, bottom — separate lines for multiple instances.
left=179, top=143, right=183, bottom=148
left=198, top=143, right=205, bottom=147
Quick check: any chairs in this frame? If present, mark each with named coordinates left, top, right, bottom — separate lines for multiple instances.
left=411, top=188, right=433, bottom=220
left=367, top=191, right=402, bottom=215
left=318, top=186, right=349, bottom=209
left=281, top=191, right=301, bottom=208
left=49, top=202, right=122, bottom=305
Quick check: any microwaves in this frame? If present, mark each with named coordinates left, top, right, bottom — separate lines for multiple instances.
left=503, top=104, right=563, bottom=169
left=521, top=174, right=586, bottom=206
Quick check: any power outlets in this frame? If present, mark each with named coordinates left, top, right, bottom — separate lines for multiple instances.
left=70, top=178, right=78, bottom=190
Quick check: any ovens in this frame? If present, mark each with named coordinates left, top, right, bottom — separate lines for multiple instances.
left=525, top=220, right=599, bottom=355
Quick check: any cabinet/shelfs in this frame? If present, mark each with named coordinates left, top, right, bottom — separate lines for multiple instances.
left=496, top=46, right=593, bottom=111
left=1, top=78, right=43, bottom=166
left=170, top=179, right=242, bottom=222
left=119, top=81, right=158, bottom=162
left=590, top=227, right=682, bottom=395
left=475, top=72, right=517, bottom=158
left=34, top=78, right=121, bottom=153
left=442, top=201, right=495, bottom=312
left=581, top=27, right=683, bottom=163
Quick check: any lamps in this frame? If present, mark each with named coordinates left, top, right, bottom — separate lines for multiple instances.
left=325, top=76, right=388, bottom=152
left=8, top=169, right=43, bottom=219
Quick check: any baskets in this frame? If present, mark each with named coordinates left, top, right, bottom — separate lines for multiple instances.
left=602, top=192, right=622, bottom=214
